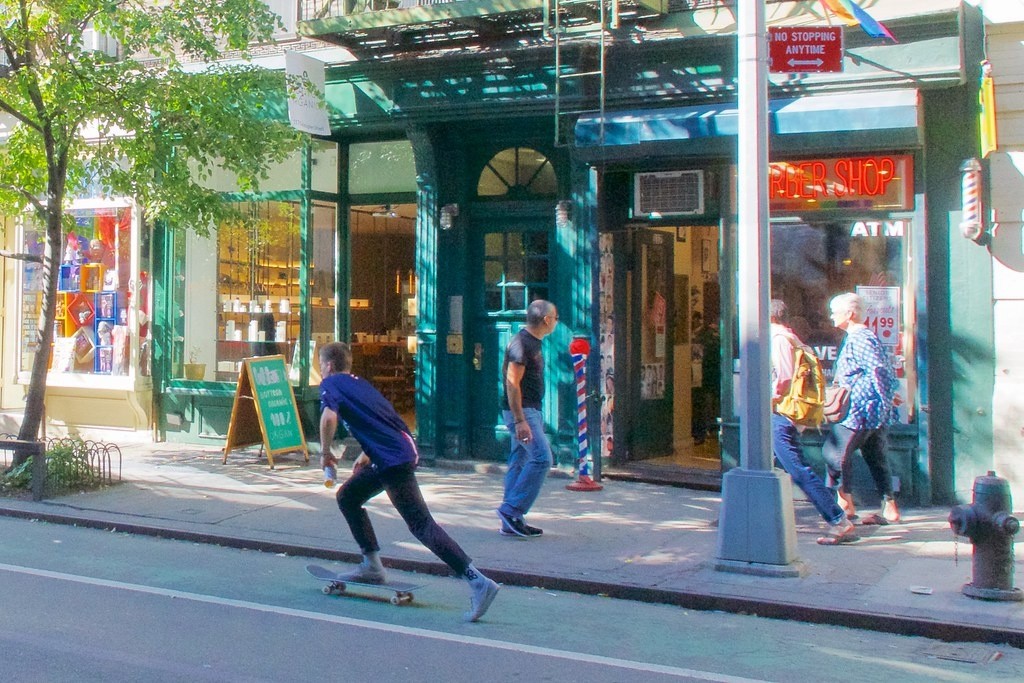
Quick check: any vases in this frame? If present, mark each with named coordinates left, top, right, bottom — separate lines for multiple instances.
left=184, top=363, right=207, bottom=380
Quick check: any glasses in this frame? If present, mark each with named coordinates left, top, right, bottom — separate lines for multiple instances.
left=548, top=313, right=560, bottom=320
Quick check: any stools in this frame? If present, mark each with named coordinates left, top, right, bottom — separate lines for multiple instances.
left=372, top=374, right=405, bottom=405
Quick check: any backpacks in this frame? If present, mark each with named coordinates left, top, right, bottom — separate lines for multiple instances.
left=771, top=333, right=828, bottom=437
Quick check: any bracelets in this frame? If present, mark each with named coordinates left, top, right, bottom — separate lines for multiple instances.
left=319, top=450, right=331, bottom=457
left=514, top=419, right=526, bottom=424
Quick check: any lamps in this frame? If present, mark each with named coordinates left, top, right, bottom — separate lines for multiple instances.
left=439, top=202, right=459, bottom=233
left=555, top=199, right=574, bottom=229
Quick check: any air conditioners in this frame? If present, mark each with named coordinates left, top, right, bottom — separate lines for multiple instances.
left=630, top=169, right=717, bottom=221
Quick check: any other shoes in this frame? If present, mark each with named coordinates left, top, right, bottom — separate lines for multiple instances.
left=499, top=521, right=543, bottom=537
left=496, top=508, right=531, bottom=538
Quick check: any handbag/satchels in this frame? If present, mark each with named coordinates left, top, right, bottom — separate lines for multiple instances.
left=822, top=384, right=851, bottom=424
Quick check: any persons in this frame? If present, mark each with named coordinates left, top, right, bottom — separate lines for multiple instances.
left=600, top=237, right=666, bottom=456
left=317, top=341, right=500, bottom=623
left=692, top=311, right=722, bottom=446
left=494, top=300, right=559, bottom=538
left=768, top=299, right=854, bottom=545
left=821, top=292, right=903, bottom=525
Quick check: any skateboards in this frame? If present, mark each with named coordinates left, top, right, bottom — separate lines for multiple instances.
left=305, top=563, right=432, bottom=606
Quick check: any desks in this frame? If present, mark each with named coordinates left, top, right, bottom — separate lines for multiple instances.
left=353, top=340, right=409, bottom=349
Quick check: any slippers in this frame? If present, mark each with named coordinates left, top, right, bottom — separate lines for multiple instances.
left=709, top=518, right=719, bottom=529
left=817, top=514, right=859, bottom=524
left=861, top=512, right=900, bottom=526
left=817, top=531, right=860, bottom=546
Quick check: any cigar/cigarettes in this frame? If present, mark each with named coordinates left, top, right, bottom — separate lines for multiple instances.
left=523, top=437, right=528, bottom=441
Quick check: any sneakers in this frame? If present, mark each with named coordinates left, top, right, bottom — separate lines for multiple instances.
left=337, top=561, right=391, bottom=585
left=465, top=579, right=501, bottom=622
